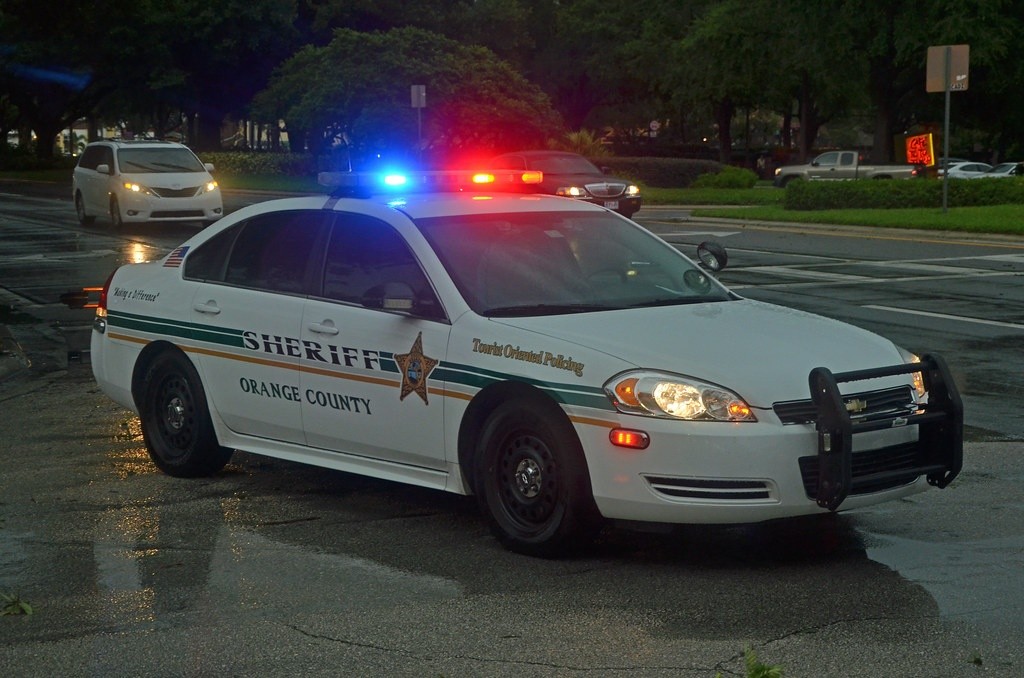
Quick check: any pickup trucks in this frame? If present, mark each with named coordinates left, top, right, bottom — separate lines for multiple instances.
left=775, top=151, right=920, bottom=191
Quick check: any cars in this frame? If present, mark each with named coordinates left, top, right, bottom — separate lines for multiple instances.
left=456, top=151, right=640, bottom=221
left=90, top=167, right=965, bottom=557
left=938, top=158, right=969, bottom=169
left=971, top=162, right=1023, bottom=177
left=937, top=162, right=993, bottom=179
left=72, top=140, right=223, bottom=230
